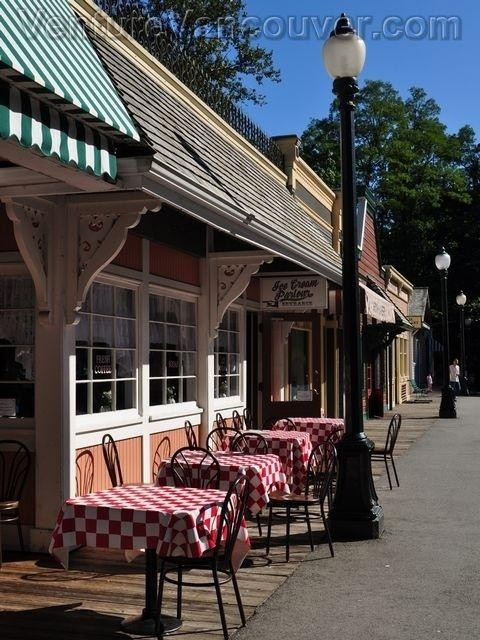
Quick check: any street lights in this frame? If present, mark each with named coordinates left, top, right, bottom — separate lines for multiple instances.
left=322, top=11, right=382, bottom=540
left=435, top=247, right=458, bottom=418
left=456, top=289, right=469, bottom=397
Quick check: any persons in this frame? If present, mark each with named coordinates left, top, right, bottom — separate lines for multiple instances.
left=426, top=372, right=434, bottom=392
left=448, top=357, right=460, bottom=406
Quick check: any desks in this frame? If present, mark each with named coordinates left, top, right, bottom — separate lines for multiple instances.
left=50, top=486, right=251, bottom=637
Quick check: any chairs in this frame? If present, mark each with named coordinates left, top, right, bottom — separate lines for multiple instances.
left=156, top=407, right=357, bottom=562
left=0, top=439, right=32, bottom=553
left=101, top=433, right=124, bottom=486
left=410, top=377, right=434, bottom=404
left=370, top=413, right=402, bottom=491
left=157, top=475, right=249, bottom=639
